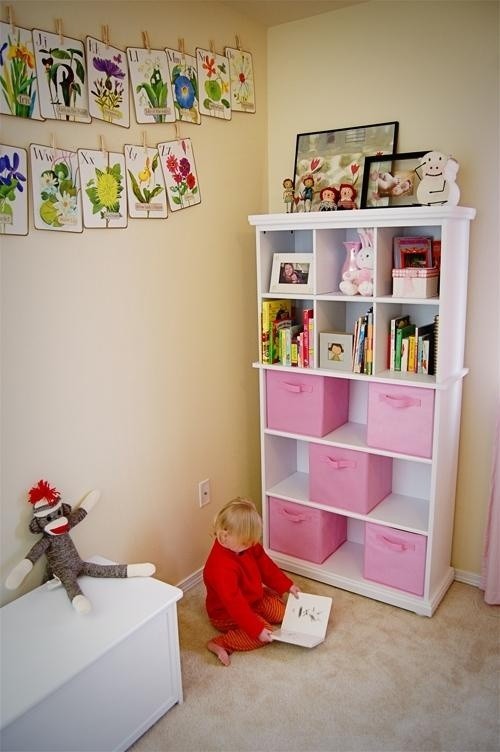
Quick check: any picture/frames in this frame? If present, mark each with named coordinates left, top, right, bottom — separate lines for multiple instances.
left=360, top=150, right=433, bottom=208
left=319, top=333, right=353, bottom=371
left=291, top=120, right=400, bottom=211
left=269, top=252, right=313, bottom=294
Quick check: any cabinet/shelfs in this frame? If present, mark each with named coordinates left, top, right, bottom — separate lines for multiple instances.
left=247, top=205, right=476, bottom=619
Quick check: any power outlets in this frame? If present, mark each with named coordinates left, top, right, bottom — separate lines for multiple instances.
left=199, top=478, right=211, bottom=507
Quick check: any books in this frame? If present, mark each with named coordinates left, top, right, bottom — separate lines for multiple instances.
left=262, top=299, right=315, bottom=369
left=351, top=307, right=373, bottom=375
left=389, top=315, right=439, bottom=378
left=268, top=592, right=333, bottom=650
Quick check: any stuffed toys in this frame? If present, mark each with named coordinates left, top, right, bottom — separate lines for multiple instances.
left=339, top=227, right=374, bottom=296
left=4, top=480, right=157, bottom=615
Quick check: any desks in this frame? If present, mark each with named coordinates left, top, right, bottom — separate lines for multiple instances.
left=0, top=556, right=184, bottom=752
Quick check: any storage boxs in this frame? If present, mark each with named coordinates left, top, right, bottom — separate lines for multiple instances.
left=269, top=497, right=347, bottom=565
left=265, top=369, right=349, bottom=438
left=309, top=442, right=392, bottom=515
left=364, top=522, right=427, bottom=596
left=366, top=381, right=435, bottom=459
left=392, top=267, right=438, bottom=299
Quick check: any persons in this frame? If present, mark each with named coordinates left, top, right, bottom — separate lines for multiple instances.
left=204, top=497, right=302, bottom=666
left=338, top=182, right=358, bottom=209
left=278, top=263, right=309, bottom=284
left=318, top=187, right=339, bottom=212
left=299, top=174, right=314, bottom=211
left=282, top=179, right=296, bottom=213
